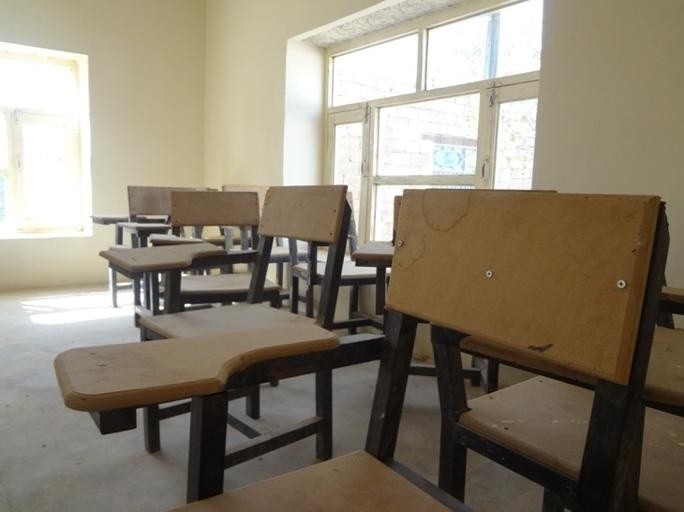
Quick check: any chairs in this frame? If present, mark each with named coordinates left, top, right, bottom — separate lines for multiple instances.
left=429, top=326, right=683, bottom=510
left=173, top=187, right=670, bottom=510
left=54, top=184, right=351, bottom=500
left=456, top=326, right=683, bottom=413
left=84, top=182, right=390, bottom=334
